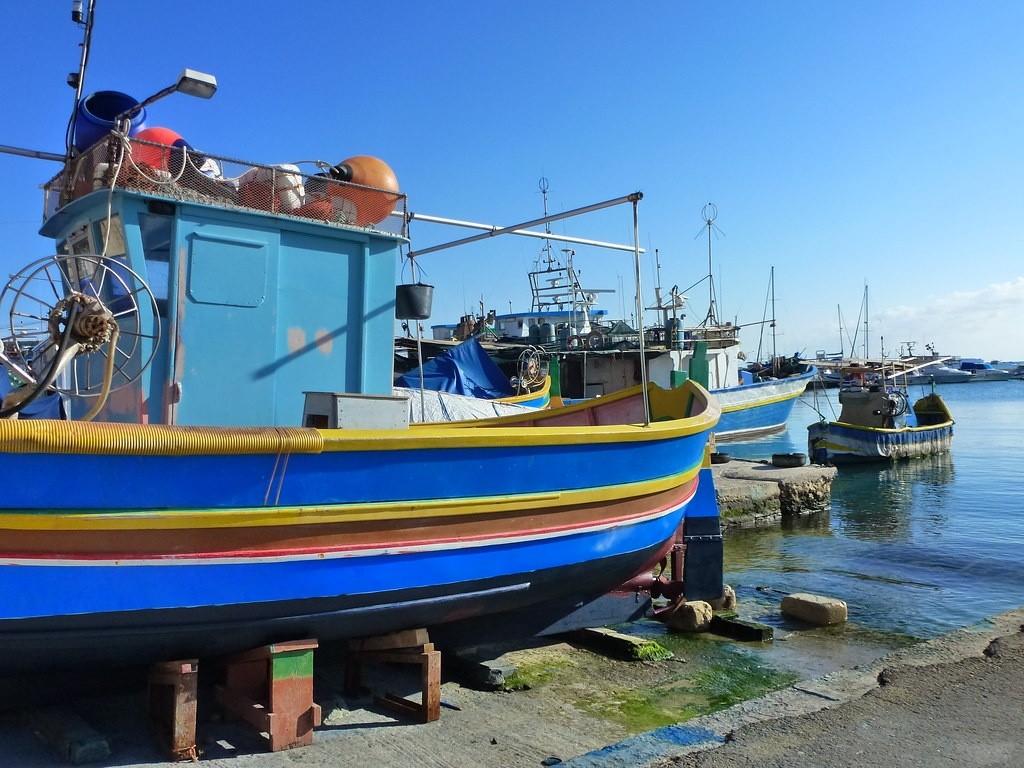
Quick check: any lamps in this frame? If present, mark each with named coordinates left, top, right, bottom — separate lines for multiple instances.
left=175, top=69, right=217, bottom=100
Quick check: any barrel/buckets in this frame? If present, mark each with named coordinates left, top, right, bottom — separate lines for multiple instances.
left=527, top=323, right=577, bottom=347
left=665, top=318, right=684, bottom=349
left=74, top=89, right=147, bottom=152
left=396, top=257, right=434, bottom=320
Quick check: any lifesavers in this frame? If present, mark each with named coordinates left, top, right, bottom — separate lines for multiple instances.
left=709, top=452, right=730, bottom=465
left=772, top=453, right=807, bottom=469
left=566, top=335, right=584, bottom=350
left=586, top=331, right=604, bottom=350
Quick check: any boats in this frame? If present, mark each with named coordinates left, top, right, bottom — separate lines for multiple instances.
left=397, top=173, right=619, bottom=382
left=794, top=283, right=957, bottom=467
left=394, top=200, right=820, bottom=446
left=806, top=302, right=1024, bottom=389
left=0, top=1, right=721, bottom=683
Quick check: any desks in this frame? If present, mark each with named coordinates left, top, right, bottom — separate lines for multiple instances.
left=302, top=389, right=411, bottom=430
